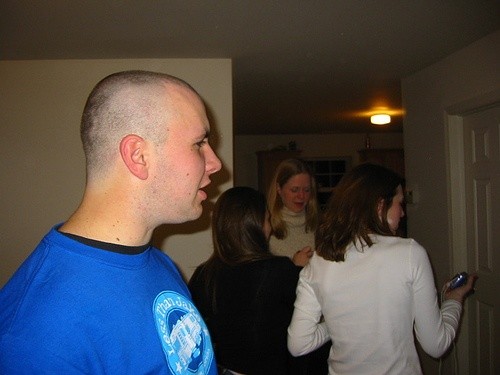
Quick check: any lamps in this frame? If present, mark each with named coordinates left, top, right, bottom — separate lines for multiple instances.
left=370, top=114, right=392, bottom=125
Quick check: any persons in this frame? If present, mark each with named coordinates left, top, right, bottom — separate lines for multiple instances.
left=1, top=70, right=223, bottom=375
left=287, top=161, right=477, bottom=375
left=189, top=185, right=330, bottom=375
left=263, top=158, right=319, bottom=263
